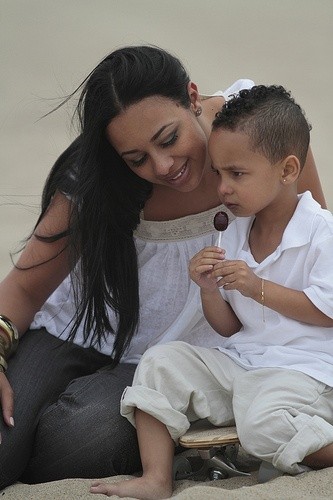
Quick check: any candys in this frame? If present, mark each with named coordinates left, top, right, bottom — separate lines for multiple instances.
left=213, top=211, right=228, bottom=232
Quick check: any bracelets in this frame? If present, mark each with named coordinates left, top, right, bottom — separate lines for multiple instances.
left=0, top=315, right=18, bottom=374
left=261, top=278, right=265, bottom=306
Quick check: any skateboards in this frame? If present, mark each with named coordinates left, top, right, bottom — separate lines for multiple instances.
left=178, top=416, right=248, bottom=480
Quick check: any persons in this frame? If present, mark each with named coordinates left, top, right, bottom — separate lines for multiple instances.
left=88, top=82, right=333, bottom=500
left=1, top=44, right=328, bottom=496
